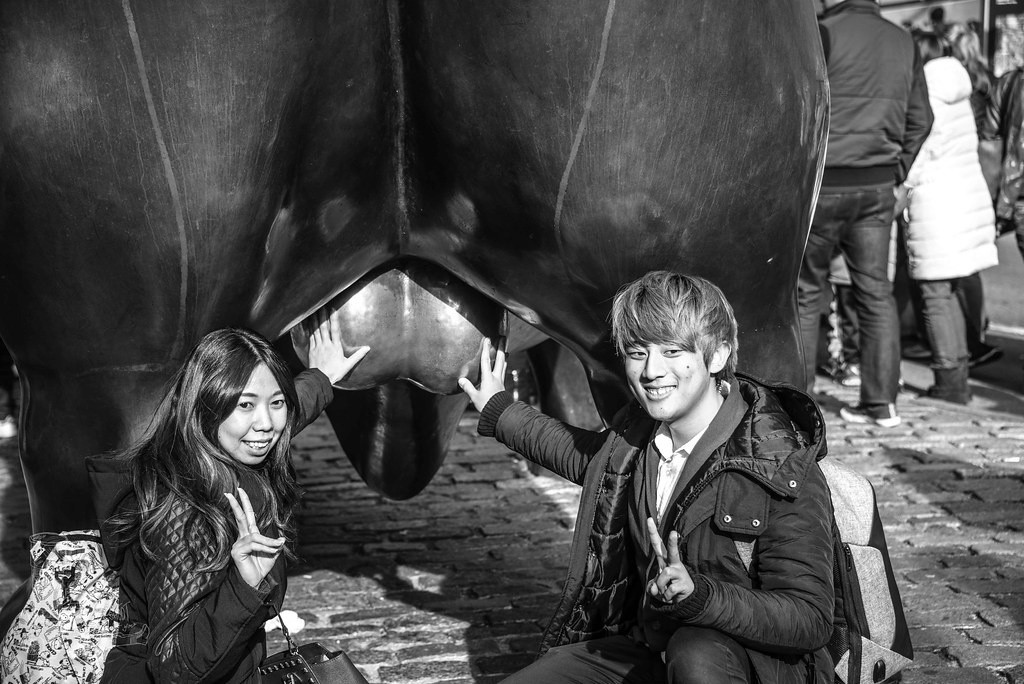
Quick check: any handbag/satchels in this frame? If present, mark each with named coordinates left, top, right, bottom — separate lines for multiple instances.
left=256, top=602, right=370, bottom=684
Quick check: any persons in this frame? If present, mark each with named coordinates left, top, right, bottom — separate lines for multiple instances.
left=458, top=270, right=839, bottom=684
left=796, top=0, right=1024, bottom=427
left=99, top=309, right=370, bottom=684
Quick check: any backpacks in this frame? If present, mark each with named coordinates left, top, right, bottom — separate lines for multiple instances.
left=0, top=529, right=119, bottom=684
left=817, top=455, right=914, bottom=684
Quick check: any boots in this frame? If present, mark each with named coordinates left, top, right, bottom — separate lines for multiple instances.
left=913, top=365, right=975, bottom=406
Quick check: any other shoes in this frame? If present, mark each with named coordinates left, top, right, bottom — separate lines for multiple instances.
left=968, top=341, right=1004, bottom=366
left=839, top=403, right=901, bottom=427
left=840, top=362, right=862, bottom=386
left=903, top=344, right=932, bottom=357
